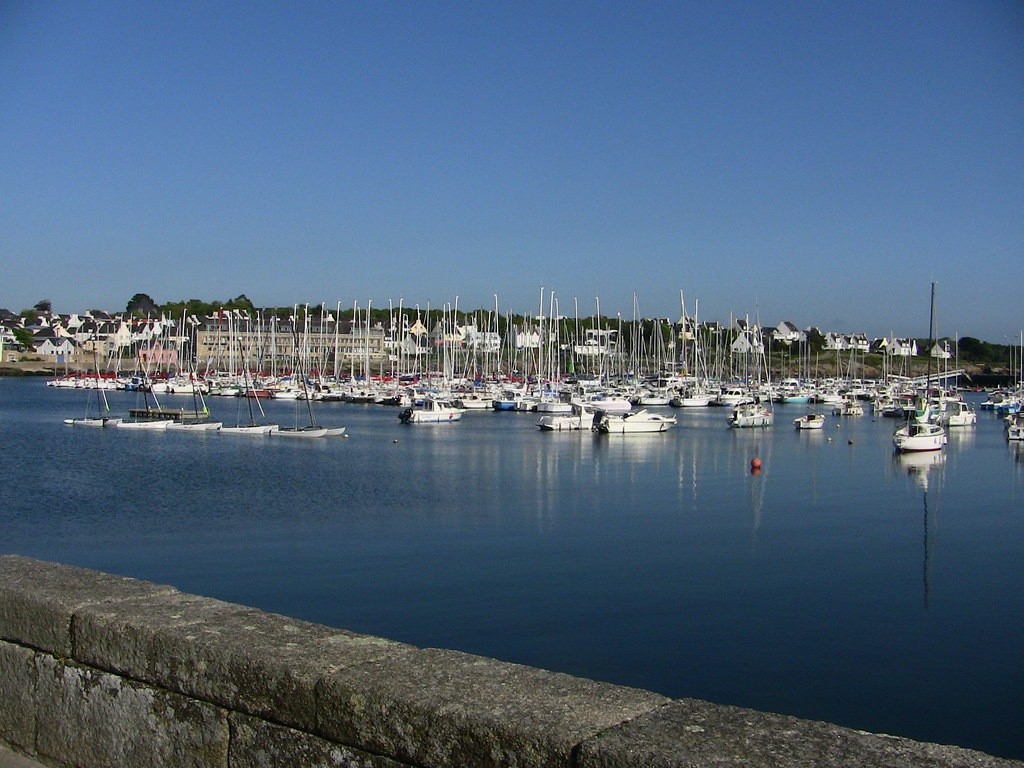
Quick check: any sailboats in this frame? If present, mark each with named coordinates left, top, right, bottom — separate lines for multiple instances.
left=45, top=281, right=1024, bottom=453
left=896, top=450, right=946, bottom=606
